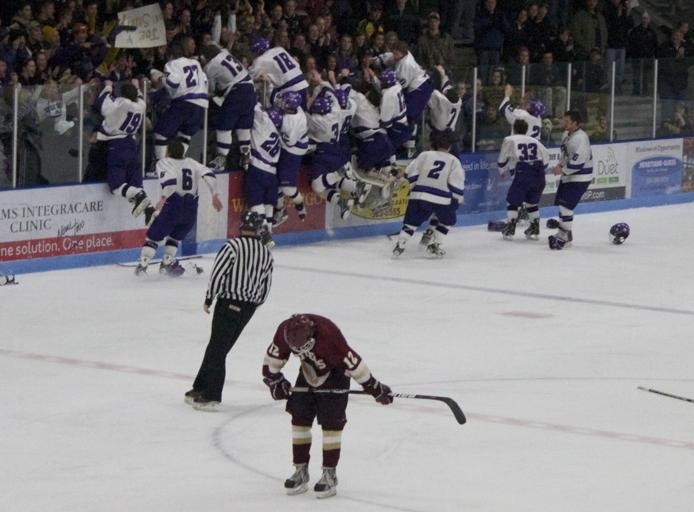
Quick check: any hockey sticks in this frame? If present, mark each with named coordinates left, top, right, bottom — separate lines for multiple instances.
left=289, top=386, right=466, bottom=424
left=111, top=256, right=203, bottom=267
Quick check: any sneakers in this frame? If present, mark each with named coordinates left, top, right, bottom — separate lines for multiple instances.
left=158, top=261, right=172, bottom=276
left=192, top=394, right=222, bottom=406
left=183, top=386, right=203, bottom=398
left=133, top=263, right=148, bottom=277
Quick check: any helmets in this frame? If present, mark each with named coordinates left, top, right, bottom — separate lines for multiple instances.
left=334, top=88, right=349, bottom=110
left=281, top=312, right=317, bottom=354
left=237, top=209, right=265, bottom=231
left=525, top=97, right=547, bottom=118
left=265, top=105, right=284, bottom=130
left=280, top=89, right=304, bottom=111
left=247, top=35, right=271, bottom=57
left=378, top=67, right=398, bottom=88
left=310, top=95, right=332, bottom=115
left=609, top=222, right=630, bottom=245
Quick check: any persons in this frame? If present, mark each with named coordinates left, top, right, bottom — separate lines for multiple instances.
left=182, top=211, right=274, bottom=410
left=262, top=312, right=393, bottom=499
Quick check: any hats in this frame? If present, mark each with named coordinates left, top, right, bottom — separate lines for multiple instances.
left=426, top=11, right=441, bottom=19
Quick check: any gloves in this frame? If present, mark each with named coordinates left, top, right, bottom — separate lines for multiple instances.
left=261, top=371, right=293, bottom=402
left=360, top=371, right=394, bottom=406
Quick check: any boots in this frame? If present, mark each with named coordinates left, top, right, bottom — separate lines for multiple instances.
left=294, top=201, right=306, bottom=220
left=236, top=146, right=251, bottom=168
left=271, top=206, right=288, bottom=225
left=205, top=154, right=228, bottom=169
left=427, top=241, right=447, bottom=256
left=500, top=221, right=517, bottom=237
left=419, top=229, right=434, bottom=247
left=283, top=462, right=310, bottom=488
left=391, top=238, right=408, bottom=256
left=548, top=226, right=573, bottom=246
left=259, top=232, right=273, bottom=247
left=130, top=190, right=148, bottom=216
left=142, top=202, right=156, bottom=227
left=312, top=466, right=339, bottom=492
left=336, top=197, right=350, bottom=219
left=523, top=222, right=541, bottom=235
left=352, top=181, right=367, bottom=205
